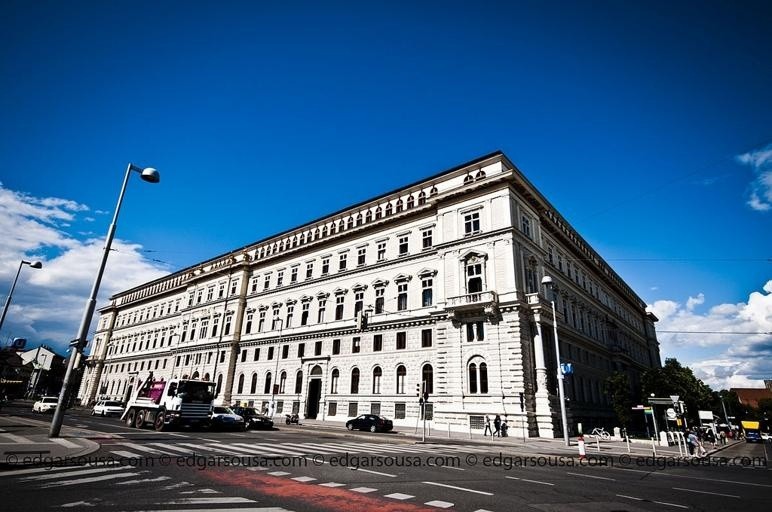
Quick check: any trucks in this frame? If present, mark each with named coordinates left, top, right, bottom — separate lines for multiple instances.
left=739, top=420, right=763, bottom=442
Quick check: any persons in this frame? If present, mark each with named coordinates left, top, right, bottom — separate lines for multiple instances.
left=492, top=414, right=501, bottom=438
left=263, top=402, right=269, bottom=416
left=685, top=425, right=727, bottom=458
left=734, top=429, right=739, bottom=440
left=484, top=414, right=493, bottom=437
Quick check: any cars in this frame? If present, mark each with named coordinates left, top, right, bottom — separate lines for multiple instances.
left=701, top=423, right=772, bottom=444
left=28, top=394, right=276, bottom=432
left=344, top=413, right=394, bottom=433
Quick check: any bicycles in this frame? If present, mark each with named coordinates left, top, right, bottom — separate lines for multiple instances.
left=588, top=424, right=612, bottom=440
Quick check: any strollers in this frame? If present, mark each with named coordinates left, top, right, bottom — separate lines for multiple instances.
left=696, top=442, right=711, bottom=461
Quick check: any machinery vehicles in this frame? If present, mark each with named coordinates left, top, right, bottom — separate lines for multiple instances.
left=120, top=367, right=217, bottom=431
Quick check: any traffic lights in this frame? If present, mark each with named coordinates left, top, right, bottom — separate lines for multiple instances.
left=673, top=403, right=680, bottom=414
left=356, top=311, right=366, bottom=331
left=77, top=353, right=89, bottom=371
left=416, top=382, right=423, bottom=399
left=680, top=401, right=689, bottom=414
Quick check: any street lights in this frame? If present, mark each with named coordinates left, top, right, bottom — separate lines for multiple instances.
left=719, top=395, right=729, bottom=423
left=46, top=160, right=162, bottom=438
left=269, top=314, right=284, bottom=417
left=540, top=272, right=571, bottom=446
left=0, top=258, right=43, bottom=330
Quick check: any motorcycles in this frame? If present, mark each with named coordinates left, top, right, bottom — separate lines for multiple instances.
left=284, top=413, right=299, bottom=425
